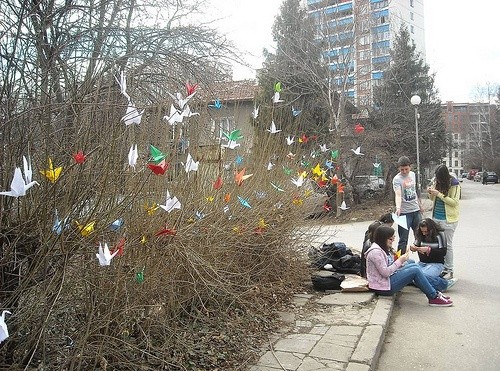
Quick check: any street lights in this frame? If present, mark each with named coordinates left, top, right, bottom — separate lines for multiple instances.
left=411, top=94, right=422, bottom=197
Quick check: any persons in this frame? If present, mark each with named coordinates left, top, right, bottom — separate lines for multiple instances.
left=482, top=169, right=488, bottom=185
left=376, top=213, right=416, bottom=280
left=428, top=165, right=461, bottom=280
left=361, top=221, right=381, bottom=277
left=410, top=218, right=447, bottom=285
left=393, top=156, right=424, bottom=257
left=364, top=225, right=453, bottom=306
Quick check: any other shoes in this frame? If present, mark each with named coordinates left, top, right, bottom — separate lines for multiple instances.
left=441, top=277, right=457, bottom=292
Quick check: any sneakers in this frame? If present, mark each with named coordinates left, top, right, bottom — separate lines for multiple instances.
left=429, top=291, right=453, bottom=307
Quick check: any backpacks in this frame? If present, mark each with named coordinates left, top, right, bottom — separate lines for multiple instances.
left=309, top=242, right=361, bottom=274
left=311, top=270, right=345, bottom=292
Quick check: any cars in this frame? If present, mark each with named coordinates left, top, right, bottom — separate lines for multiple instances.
left=461, top=169, right=498, bottom=183
left=354, top=175, right=386, bottom=200
